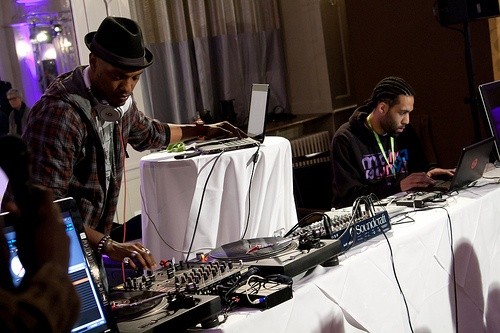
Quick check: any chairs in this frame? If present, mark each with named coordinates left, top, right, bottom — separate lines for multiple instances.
left=292, top=150, right=337, bottom=228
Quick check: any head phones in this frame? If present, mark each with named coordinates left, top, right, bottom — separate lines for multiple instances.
left=82, top=66, right=123, bottom=122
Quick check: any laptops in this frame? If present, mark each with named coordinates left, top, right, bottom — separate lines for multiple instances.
left=408, top=137, right=495, bottom=192
left=196, top=83, right=270, bottom=155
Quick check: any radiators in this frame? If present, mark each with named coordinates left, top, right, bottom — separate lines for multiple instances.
left=291, top=130, right=332, bottom=167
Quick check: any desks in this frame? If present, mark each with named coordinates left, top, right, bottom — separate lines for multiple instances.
left=138, top=136, right=299, bottom=270
left=188, top=167, right=500, bottom=333
left=262, top=113, right=333, bottom=136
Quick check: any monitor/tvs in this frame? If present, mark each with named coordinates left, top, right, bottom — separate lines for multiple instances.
left=0, top=196, right=118, bottom=333
left=478, top=80, right=500, bottom=161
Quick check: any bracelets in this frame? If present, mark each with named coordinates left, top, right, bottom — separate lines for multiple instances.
left=95, top=234, right=111, bottom=253
left=192, top=120, right=206, bottom=140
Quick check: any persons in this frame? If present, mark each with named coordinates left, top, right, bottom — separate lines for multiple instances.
left=21, top=15, right=248, bottom=274
left=0, top=79, right=81, bottom=333
left=330, top=77, right=456, bottom=210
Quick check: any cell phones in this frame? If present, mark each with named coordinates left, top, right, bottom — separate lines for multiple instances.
left=174, top=151, right=201, bottom=159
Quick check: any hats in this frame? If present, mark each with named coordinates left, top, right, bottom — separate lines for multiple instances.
left=84, top=15, right=154, bottom=69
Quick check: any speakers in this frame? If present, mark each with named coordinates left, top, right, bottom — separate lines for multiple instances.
left=434, top=0, right=500, bottom=25
